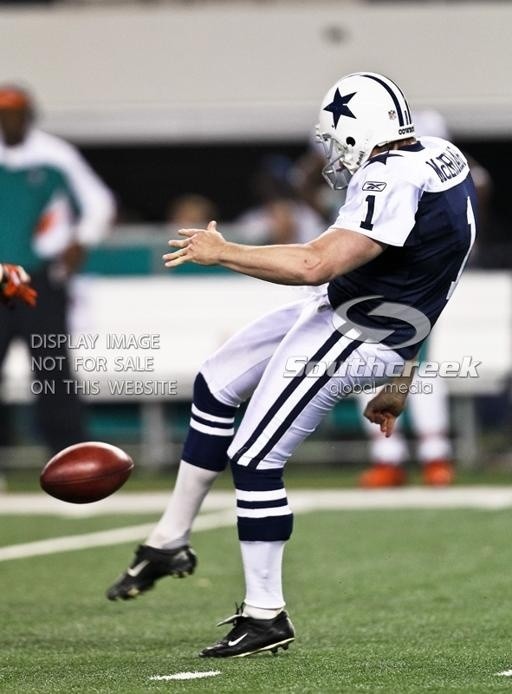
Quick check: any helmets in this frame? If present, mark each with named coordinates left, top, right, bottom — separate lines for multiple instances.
left=315, top=68, right=417, bottom=177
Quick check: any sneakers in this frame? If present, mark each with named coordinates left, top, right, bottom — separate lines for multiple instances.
left=199, top=607, right=300, bottom=661
left=104, top=538, right=201, bottom=602
left=418, top=457, right=459, bottom=488
left=356, top=457, right=412, bottom=488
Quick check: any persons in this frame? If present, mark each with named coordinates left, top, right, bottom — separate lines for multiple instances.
left=104, top=69, right=480, bottom=665
left=0, top=85, right=118, bottom=490
left=133, top=104, right=493, bottom=486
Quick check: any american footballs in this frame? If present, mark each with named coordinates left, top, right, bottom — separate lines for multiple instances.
left=40, top=441, right=134, bottom=503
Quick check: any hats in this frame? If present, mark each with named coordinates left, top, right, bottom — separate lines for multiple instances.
left=0, top=87, right=33, bottom=111
